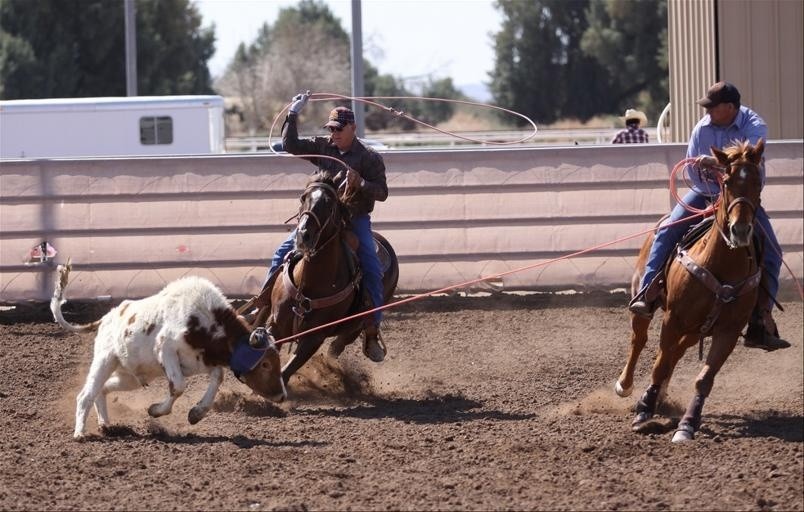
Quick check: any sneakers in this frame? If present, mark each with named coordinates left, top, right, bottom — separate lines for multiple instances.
left=367, top=337, right=385, bottom=362
left=743, top=325, right=791, bottom=349
left=631, top=292, right=651, bottom=314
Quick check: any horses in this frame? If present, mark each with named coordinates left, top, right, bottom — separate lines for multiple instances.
left=614, top=135, right=762, bottom=446
left=252, top=168, right=400, bottom=388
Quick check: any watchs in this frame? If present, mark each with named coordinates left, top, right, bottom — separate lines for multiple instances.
left=356, top=177, right=366, bottom=190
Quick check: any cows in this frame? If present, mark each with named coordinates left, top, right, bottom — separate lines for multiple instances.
left=49, top=256, right=288, bottom=443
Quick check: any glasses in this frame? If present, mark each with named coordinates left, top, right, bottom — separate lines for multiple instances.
left=328, top=127, right=343, bottom=133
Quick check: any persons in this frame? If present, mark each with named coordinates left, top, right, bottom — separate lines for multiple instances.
left=610, top=108, right=651, bottom=143
left=627, top=81, right=791, bottom=350
left=234, top=90, right=388, bottom=363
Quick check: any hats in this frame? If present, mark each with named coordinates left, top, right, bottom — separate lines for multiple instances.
left=620, top=109, right=648, bottom=127
left=695, top=81, right=741, bottom=108
left=323, top=107, right=355, bottom=128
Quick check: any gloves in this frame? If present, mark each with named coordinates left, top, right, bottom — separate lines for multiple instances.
left=289, top=90, right=311, bottom=113
left=695, top=154, right=718, bottom=168
left=345, top=168, right=362, bottom=189
